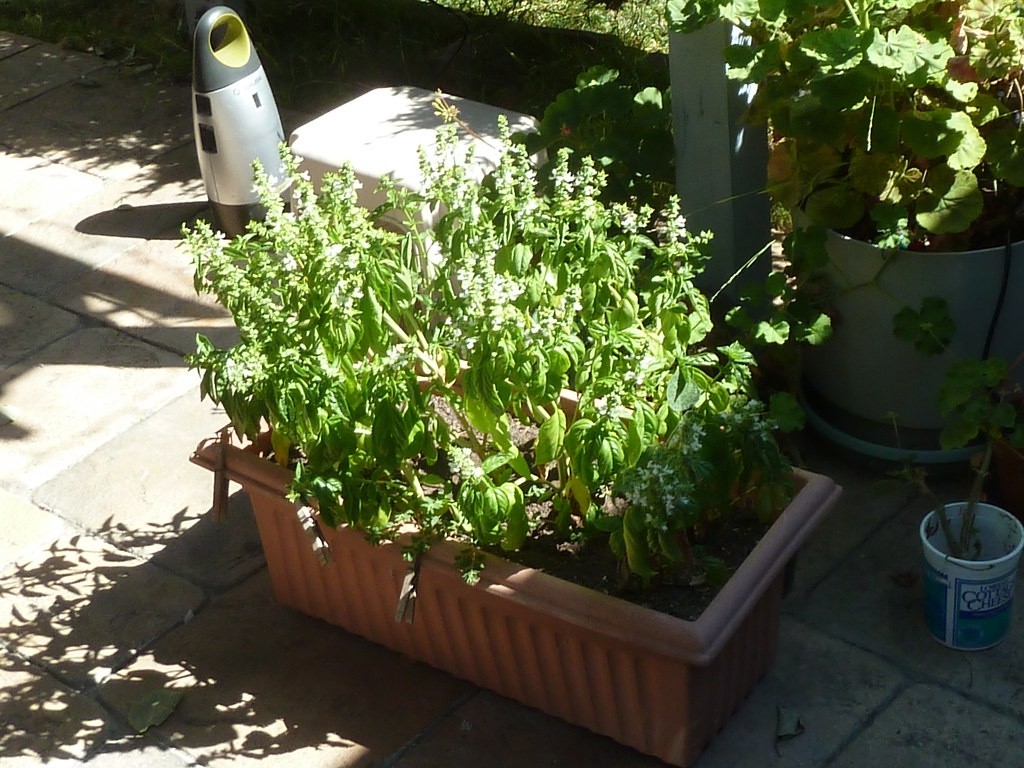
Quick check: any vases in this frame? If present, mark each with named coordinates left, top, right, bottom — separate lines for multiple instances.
left=994, top=418, right=1024, bottom=525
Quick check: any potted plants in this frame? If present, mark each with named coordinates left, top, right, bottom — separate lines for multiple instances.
left=194, top=85, right=846, bottom=767
left=656, top=0, right=1024, bottom=467
left=882, top=295, right=1024, bottom=652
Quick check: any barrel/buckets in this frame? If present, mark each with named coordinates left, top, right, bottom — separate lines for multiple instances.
left=920, top=502, right=1024, bottom=651
